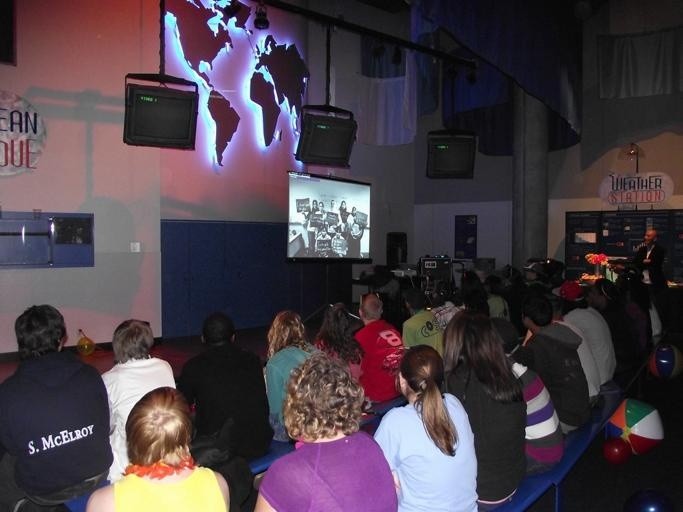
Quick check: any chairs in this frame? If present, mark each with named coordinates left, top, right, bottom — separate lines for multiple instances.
left=360, top=421, right=382, bottom=438
left=239, top=444, right=296, bottom=508
left=367, top=394, right=408, bottom=418
left=540, top=436, right=589, bottom=487
left=583, top=415, right=604, bottom=441
left=485, top=473, right=560, bottom=512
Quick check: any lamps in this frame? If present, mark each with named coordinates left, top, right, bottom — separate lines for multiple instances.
left=619, top=142, right=646, bottom=209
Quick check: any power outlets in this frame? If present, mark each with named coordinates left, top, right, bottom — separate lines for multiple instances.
left=130, top=242, right=140, bottom=253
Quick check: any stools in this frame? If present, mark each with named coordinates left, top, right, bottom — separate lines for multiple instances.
left=64, top=481, right=111, bottom=512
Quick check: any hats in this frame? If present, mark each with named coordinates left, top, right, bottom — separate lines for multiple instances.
left=553, top=280, right=585, bottom=302
left=522, top=262, right=544, bottom=275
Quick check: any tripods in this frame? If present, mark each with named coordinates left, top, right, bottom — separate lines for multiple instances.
left=302, top=265, right=361, bottom=325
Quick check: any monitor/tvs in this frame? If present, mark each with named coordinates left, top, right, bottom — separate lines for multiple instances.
left=426, top=131, right=476, bottom=179
left=294, top=104, right=357, bottom=168
left=124, top=74, right=199, bottom=150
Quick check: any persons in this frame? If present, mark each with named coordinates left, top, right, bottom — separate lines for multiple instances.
left=317, top=303, right=363, bottom=382
left=523, top=261, right=545, bottom=291
left=545, top=296, right=601, bottom=408
left=264, top=313, right=320, bottom=442
left=374, top=344, right=479, bottom=511
left=520, top=296, right=592, bottom=436
left=400, top=287, right=441, bottom=355
left=587, top=277, right=640, bottom=365
left=612, top=272, right=650, bottom=351
left=441, top=310, right=526, bottom=510
left=648, top=300, right=663, bottom=345
left=432, top=284, right=460, bottom=328
left=96, top=320, right=179, bottom=483
left=0, top=304, right=114, bottom=512
left=302, top=200, right=363, bottom=257
left=489, top=314, right=564, bottom=477
left=554, top=280, right=615, bottom=387
left=254, top=354, right=397, bottom=512
left=87, top=387, right=229, bottom=512
left=353, top=294, right=406, bottom=417
left=458, top=271, right=490, bottom=312
left=178, top=316, right=272, bottom=460
left=634, top=231, right=663, bottom=302
left=502, top=267, right=529, bottom=312
left=485, top=274, right=509, bottom=322
